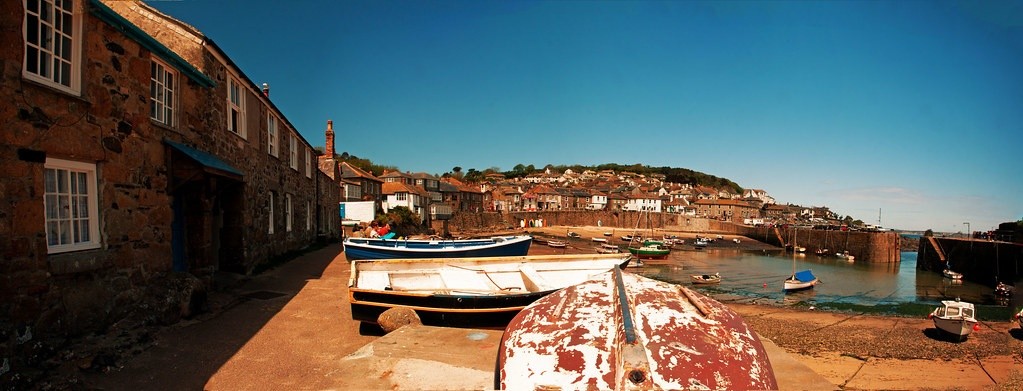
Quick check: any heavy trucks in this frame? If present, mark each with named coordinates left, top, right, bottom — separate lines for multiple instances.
left=861, top=225, right=885, bottom=233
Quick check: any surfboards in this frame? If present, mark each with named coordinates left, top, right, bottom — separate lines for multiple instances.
left=520, top=218, right=547, bottom=227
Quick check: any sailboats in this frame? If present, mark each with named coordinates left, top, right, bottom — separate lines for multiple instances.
left=629, top=191, right=670, bottom=259
left=782, top=228, right=819, bottom=291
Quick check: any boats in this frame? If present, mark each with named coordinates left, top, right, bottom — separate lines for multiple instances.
left=567, top=231, right=582, bottom=238
left=495, top=263, right=780, bottom=391
left=347, top=253, right=632, bottom=321
left=591, top=237, right=608, bottom=243
left=621, top=235, right=740, bottom=247
left=534, top=237, right=570, bottom=248
left=594, top=244, right=619, bottom=254
left=604, top=233, right=612, bottom=236
left=995, top=283, right=1017, bottom=310
left=344, top=234, right=531, bottom=261
left=520, top=218, right=547, bottom=227
left=785, top=242, right=806, bottom=252
left=943, top=269, right=963, bottom=279
left=932, top=297, right=977, bottom=336
left=814, top=232, right=855, bottom=260
left=690, top=274, right=721, bottom=284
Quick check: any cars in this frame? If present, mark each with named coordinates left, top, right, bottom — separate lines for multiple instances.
left=812, top=225, right=861, bottom=232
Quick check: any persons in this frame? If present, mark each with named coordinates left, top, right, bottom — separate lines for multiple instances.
left=986, top=231, right=991, bottom=240
left=352, top=221, right=394, bottom=239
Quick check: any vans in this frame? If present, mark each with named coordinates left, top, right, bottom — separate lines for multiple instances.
left=744, top=219, right=753, bottom=224
left=585, top=204, right=602, bottom=211
left=794, top=217, right=827, bottom=222
